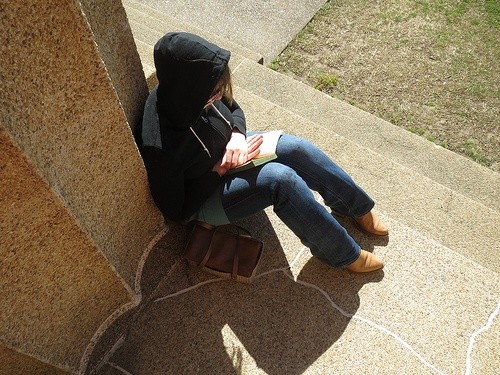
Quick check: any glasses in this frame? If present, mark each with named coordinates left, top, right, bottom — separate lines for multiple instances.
left=205, top=82, right=227, bottom=103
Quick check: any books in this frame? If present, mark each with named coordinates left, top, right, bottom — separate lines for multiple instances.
left=227, top=130, right=283, bottom=174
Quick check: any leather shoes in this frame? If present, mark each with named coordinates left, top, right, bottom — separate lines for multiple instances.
left=346, top=249, right=383, bottom=273
left=331, top=211, right=389, bottom=237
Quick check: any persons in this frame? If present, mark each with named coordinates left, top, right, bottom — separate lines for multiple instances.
left=140, top=33, right=389, bottom=274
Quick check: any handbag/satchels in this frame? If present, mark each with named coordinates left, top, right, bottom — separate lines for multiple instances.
left=186, top=222, right=263, bottom=282
